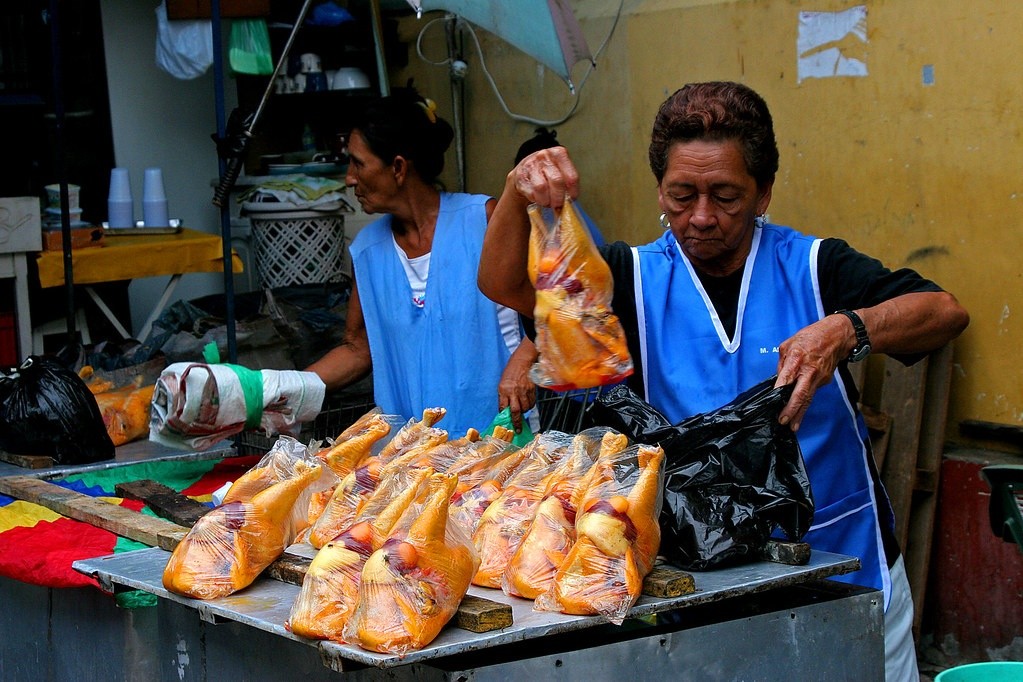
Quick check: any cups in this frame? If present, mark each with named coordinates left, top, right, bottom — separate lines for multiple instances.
left=141, top=166, right=169, bottom=229
left=107, top=167, right=134, bottom=229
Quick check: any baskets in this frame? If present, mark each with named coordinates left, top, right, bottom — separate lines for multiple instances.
left=242, top=200, right=354, bottom=289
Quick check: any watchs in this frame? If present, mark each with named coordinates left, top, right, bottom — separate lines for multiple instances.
left=834, top=308, right=872, bottom=362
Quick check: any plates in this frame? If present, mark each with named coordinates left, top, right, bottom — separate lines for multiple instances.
left=269, top=164, right=301, bottom=172
left=302, top=163, right=335, bottom=171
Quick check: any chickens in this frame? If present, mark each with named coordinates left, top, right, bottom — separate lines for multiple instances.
left=77, top=193, right=664, bottom=659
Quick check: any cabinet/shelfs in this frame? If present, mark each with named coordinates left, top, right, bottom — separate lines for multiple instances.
left=165, top=0, right=391, bottom=366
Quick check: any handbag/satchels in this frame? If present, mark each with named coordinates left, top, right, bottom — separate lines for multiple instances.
left=0, top=355, right=115, bottom=465
left=596, top=375, right=815, bottom=573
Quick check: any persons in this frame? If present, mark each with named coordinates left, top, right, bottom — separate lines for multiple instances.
left=478, top=82, right=971, bottom=682
left=304, top=96, right=540, bottom=445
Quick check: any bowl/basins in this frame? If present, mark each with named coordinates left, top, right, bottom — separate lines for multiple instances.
left=332, top=67, right=370, bottom=90
left=44, top=184, right=89, bottom=231
